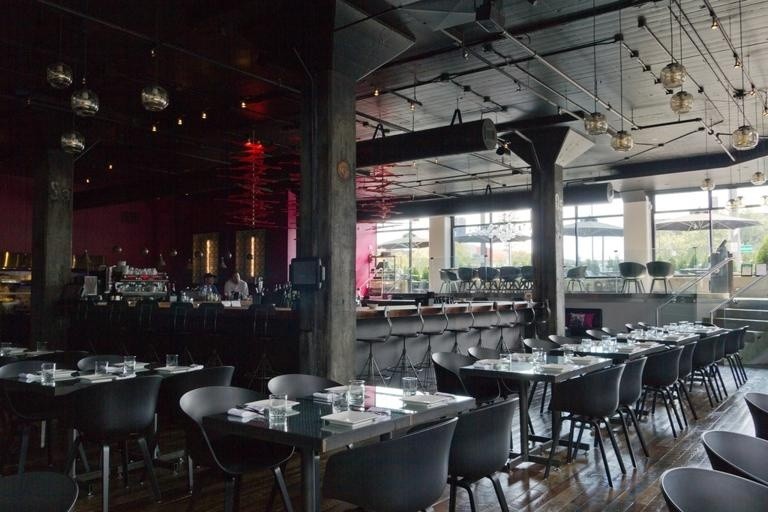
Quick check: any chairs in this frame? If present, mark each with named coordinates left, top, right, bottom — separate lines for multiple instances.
left=744, top=391, right=768, bottom=439
left=478, top=266, right=500, bottom=289
left=179, top=386, right=297, bottom=512
left=140, top=365, right=235, bottom=495
left=389, top=302, right=424, bottom=392
left=619, top=261, right=647, bottom=294
left=690, top=332, right=729, bottom=400
left=520, top=266, right=536, bottom=290
left=544, top=363, right=626, bottom=488
left=467, top=345, right=536, bottom=436
left=602, top=326, right=626, bottom=337
left=669, top=333, right=721, bottom=408
left=573, top=356, right=649, bottom=468
left=318, top=417, right=460, bottom=511
left=510, top=302, right=536, bottom=353
left=565, top=265, right=588, bottom=292
left=431, top=351, right=514, bottom=451
left=75, top=353, right=160, bottom=489
left=406, top=397, right=520, bottom=511
left=625, top=345, right=686, bottom=439
left=586, top=329, right=611, bottom=340
left=499, top=266, right=521, bottom=290
left=55, top=375, right=162, bottom=512
left=492, top=303, right=519, bottom=353
left=0, top=360, right=74, bottom=472
left=660, top=465, right=768, bottom=512
left=709, top=325, right=750, bottom=384
left=356, top=307, right=393, bottom=386
left=533, top=299, right=551, bottom=339
left=416, top=302, right=449, bottom=391
left=43, top=295, right=282, bottom=373
left=445, top=298, right=475, bottom=353
left=646, top=261, right=672, bottom=297
left=548, top=333, right=581, bottom=348
left=268, top=373, right=353, bottom=511
left=625, top=322, right=647, bottom=332
left=639, top=342, right=698, bottom=426
left=708, top=328, right=742, bottom=390
left=0, top=469, right=79, bottom=512
left=469, top=302, right=501, bottom=353
left=523, top=338, right=562, bottom=415
left=637, top=320, right=657, bottom=330
left=439, top=268, right=457, bottom=293
left=458, top=267, right=476, bottom=293
left=703, top=428, right=768, bottom=484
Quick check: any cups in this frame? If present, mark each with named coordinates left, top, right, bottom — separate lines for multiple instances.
left=349, top=380, right=365, bottom=411
left=402, top=376, right=418, bottom=398
left=534, top=352, right=546, bottom=372
left=499, top=353, right=511, bottom=372
left=687, top=323, right=694, bottom=328
left=695, top=321, right=704, bottom=329
left=563, top=344, right=574, bottom=363
left=656, top=328, right=663, bottom=336
left=94, top=361, right=108, bottom=376
left=669, top=323, right=676, bottom=332
left=678, top=320, right=689, bottom=334
left=532, top=347, right=544, bottom=359
left=269, top=394, right=288, bottom=432
left=122, top=355, right=136, bottom=376
left=40, top=362, right=56, bottom=387
left=602, top=335, right=610, bottom=351
left=331, top=390, right=349, bottom=414
left=582, top=339, right=592, bottom=353
left=608, top=337, right=617, bottom=352
left=627, top=334, right=636, bottom=347
left=166, top=354, right=179, bottom=370
left=632, top=331, right=641, bottom=344
left=635, top=329, right=643, bottom=336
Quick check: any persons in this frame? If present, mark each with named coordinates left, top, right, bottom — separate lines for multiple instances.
left=197, top=275, right=219, bottom=295
left=223, top=271, right=249, bottom=300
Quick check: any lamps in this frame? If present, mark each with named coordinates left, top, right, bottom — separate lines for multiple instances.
left=700, top=100, right=715, bottom=193
left=585, top=1, right=608, bottom=135
left=141, top=84, right=169, bottom=113
left=69, top=90, right=101, bottom=117
left=751, top=157, right=766, bottom=186
left=726, top=166, right=739, bottom=212
left=728, top=0, right=759, bottom=151
left=46, top=63, right=73, bottom=90
left=60, top=129, right=86, bottom=156
left=611, top=8, right=633, bottom=151
left=669, top=1, right=695, bottom=113
left=660, top=0, right=687, bottom=88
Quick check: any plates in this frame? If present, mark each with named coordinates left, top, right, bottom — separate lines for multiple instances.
left=54, top=372, right=74, bottom=380
left=320, top=423, right=346, bottom=438
left=476, top=359, right=497, bottom=365
left=245, top=398, right=300, bottom=411
left=541, top=363, right=562, bottom=370
left=638, top=343, right=651, bottom=348
left=400, top=392, right=451, bottom=406
left=320, top=409, right=376, bottom=426
left=618, top=346, right=632, bottom=350
left=570, top=355, right=591, bottom=363
left=156, top=365, right=189, bottom=373
left=115, top=361, right=149, bottom=372
left=324, top=383, right=349, bottom=394
